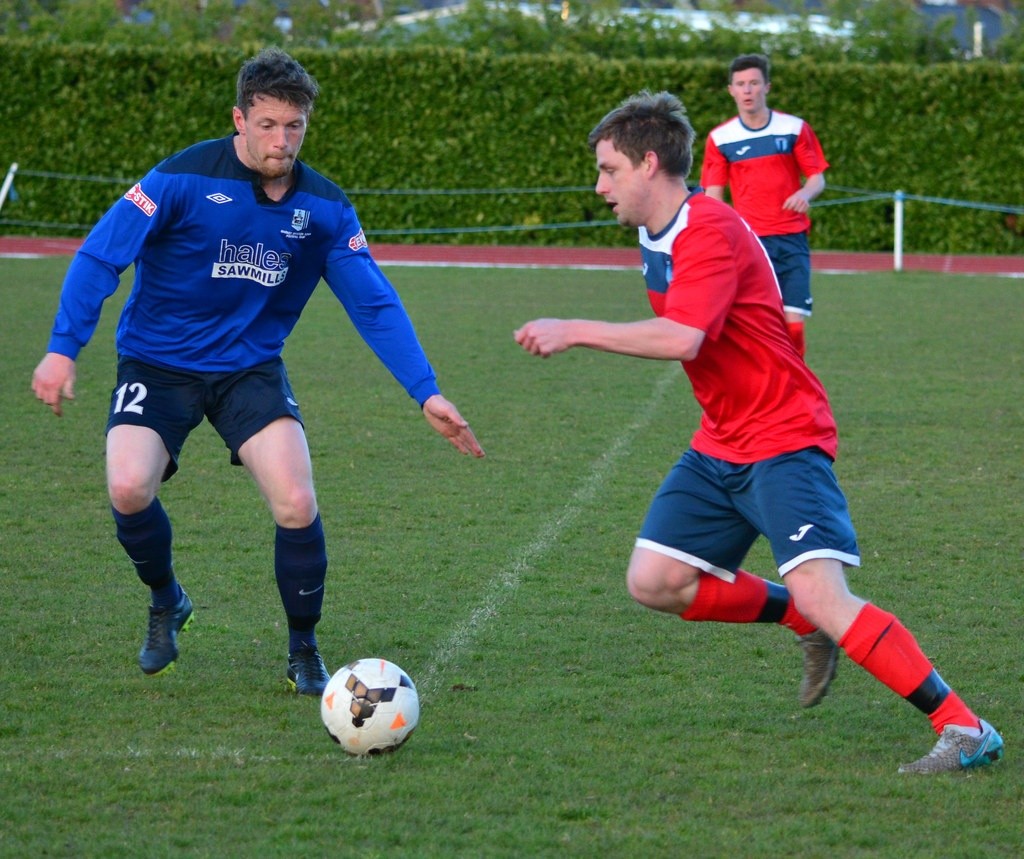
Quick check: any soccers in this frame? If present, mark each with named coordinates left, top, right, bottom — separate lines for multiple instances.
left=321, top=658, right=421, bottom=755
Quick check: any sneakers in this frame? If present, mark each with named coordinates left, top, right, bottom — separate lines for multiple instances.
left=898, top=718, right=1004, bottom=774
left=138, top=584, right=194, bottom=676
left=287, top=643, right=331, bottom=696
left=797, top=629, right=840, bottom=709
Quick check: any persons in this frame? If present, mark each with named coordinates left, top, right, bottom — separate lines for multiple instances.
left=29, top=46, right=484, bottom=697
left=513, top=53, right=1004, bottom=776
left=699, top=54, right=829, bottom=332
